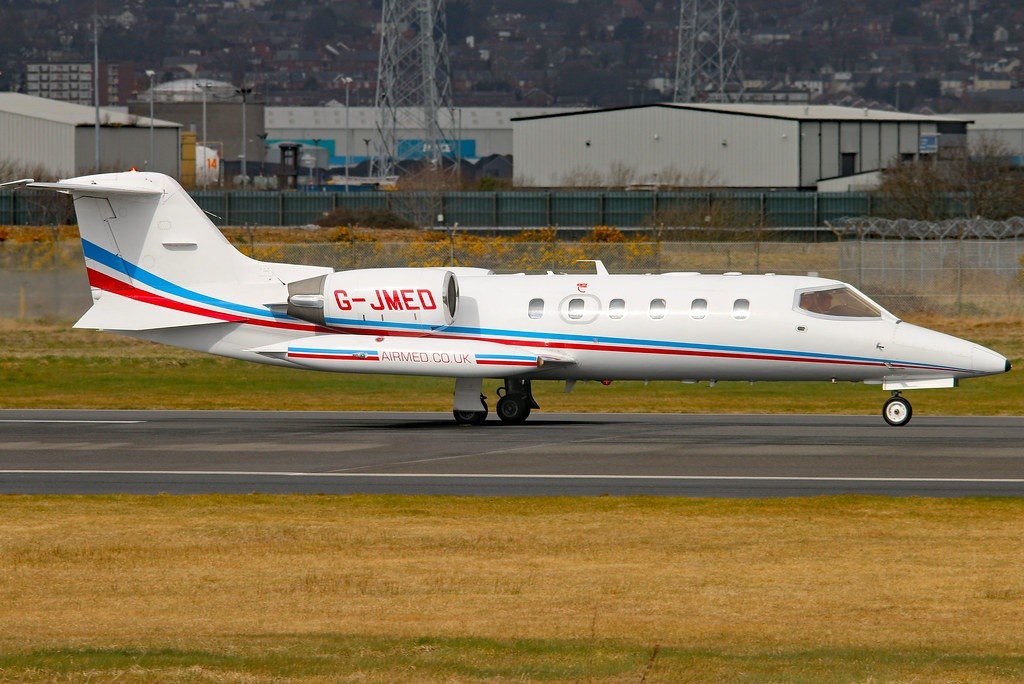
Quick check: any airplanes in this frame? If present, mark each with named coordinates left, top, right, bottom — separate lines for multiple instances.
left=25, top=167, right=1013, bottom=427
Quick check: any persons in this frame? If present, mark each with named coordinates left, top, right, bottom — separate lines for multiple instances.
left=808, top=292, right=833, bottom=312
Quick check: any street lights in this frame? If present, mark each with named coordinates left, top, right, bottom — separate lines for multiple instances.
left=145, top=70, right=156, bottom=169
left=196, top=83, right=213, bottom=192
left=236, top=89, right=252, bottom=190
left=342, top=77, right=353, bottom=192
